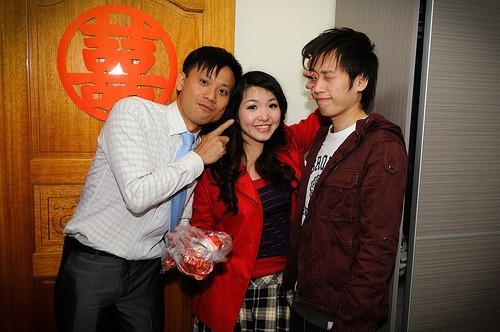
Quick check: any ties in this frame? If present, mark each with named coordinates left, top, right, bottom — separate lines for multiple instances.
left=170, top=132, right=195, bottom=232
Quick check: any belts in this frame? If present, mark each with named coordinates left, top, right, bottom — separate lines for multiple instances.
left=64, top=235, right=120, bottom=257
left=291, top=300, right=337, bottom=331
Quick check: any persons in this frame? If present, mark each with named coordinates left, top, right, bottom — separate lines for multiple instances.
left=167, top=70, right=324, bottom=332
left=279, top=27, right=408, bottom=332
left=53, top=45, right=243, bottom=332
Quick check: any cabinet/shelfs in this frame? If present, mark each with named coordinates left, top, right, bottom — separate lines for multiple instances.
left=335, top=0, right=500, bottom=332
